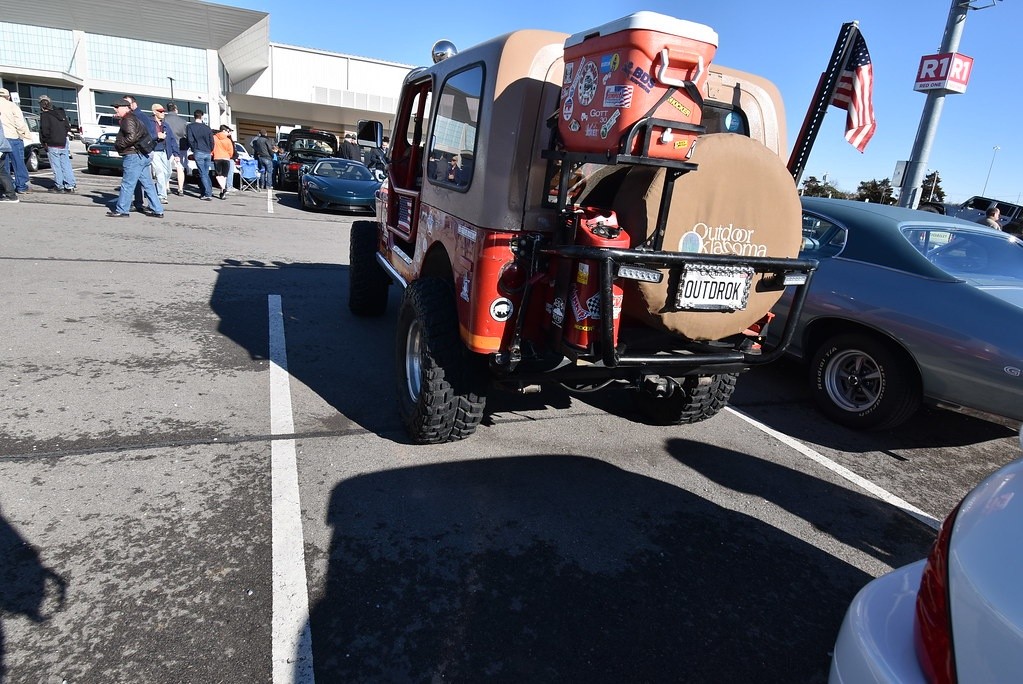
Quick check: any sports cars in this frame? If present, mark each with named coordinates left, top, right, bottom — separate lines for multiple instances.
left=300, top=158, right=382, bottom=214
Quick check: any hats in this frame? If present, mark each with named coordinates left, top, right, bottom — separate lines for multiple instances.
left=0, top=87, right=10, bottom=97
left=259, top=128, right=268, bottom=136
left=110, top=100, right=131, bottom=107
left=151, top=104, right=166, bottom=112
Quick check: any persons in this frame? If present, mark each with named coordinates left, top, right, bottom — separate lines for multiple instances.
left=149, top=104, right=179, bottom=204
left=314, top=141, right=322, bottom=149
left=0, top=88, right=30, bottom=204
left=252, top=129, right=287, bottom=189
left=428, top=153, right=461, bottom=186
left=340, top=134, right=360, bottom=161
left=38, top=95, right=77, bottom=194
left=109, top=95, right=164, bottom=218
left=164, top=102, right=187, bottom=196
left=211, top=125, right=239, bottom=199
left=976, top=208, right=1000, bottom=231
left=370, top=139, right=389, bottom=170
left=187, top=110, right=214, bottom=200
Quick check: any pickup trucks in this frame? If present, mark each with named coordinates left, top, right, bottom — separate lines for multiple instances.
left=79, top=113, right=136, bottom=152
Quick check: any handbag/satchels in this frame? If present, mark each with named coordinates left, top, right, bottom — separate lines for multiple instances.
left=180, top=137, right=190, bottom=152
left=133, top=135, right=159, bottom=158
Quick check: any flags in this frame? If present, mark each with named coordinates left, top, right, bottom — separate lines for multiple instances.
left=830, top=28, right=877, bottom=154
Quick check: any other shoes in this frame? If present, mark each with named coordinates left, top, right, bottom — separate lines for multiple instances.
left=176, top=189, right=183, bottom=196
left=106, top=210, right=129, bottom=217
left=48, top=186, right=75, bottom=194
left=128, top=204, right=145, bottom=214
left=18, top=188, right=34, bottom=194
left=219, top=189, right=229, bottom=200
left=167, top=190, right=172, bottom=195
left=0, top=194, right=20, bottom=203
left=160, top=198, right=168, bottom=205
left=200, top=194, right=213, bottom=201
left=261, top=185, right=274, bottom=189
left=145, top=212, right=165, bottom=219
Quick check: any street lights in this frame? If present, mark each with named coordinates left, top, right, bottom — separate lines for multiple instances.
left=982, top=146, right=1000, bottom=197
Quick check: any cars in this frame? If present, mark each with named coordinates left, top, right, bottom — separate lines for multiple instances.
left=745, top=197, right=1023, bottom=431
left=87, top=132, right=125, bottom=174
left=22, top=111, right=74, bottom=171
left=279, top=129, right=341, bottom=189
left=185, top=142, right=252, bottom=184
left=828, top=459, right=1023, bottom=684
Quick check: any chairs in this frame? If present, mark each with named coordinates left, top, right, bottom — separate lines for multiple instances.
left=236, top=158, right=261, bottom=193
left=318, top=164, right=338, bottom=178
left=342, top=165, right=363, bottom=179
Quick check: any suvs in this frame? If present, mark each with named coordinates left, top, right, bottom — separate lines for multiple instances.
left=348, top=27, right=819, bottom=445
left=917, top=196, right=1023, bottom=234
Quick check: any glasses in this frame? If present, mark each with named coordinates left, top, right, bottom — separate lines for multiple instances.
left=226, top=130, right=230, bottom=134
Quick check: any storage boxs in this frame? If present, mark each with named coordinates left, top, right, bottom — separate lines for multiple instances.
left=559, top=13, right=719, bottom=162
